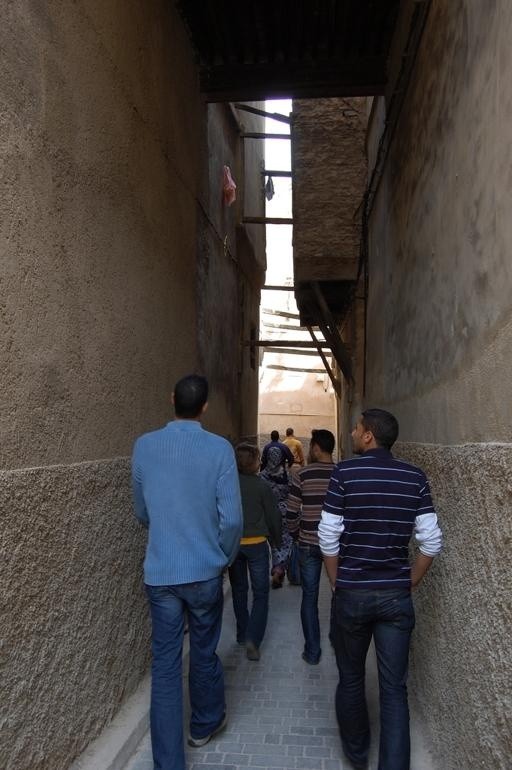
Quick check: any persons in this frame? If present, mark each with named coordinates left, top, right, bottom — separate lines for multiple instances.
left=281, top=427, right=307, bottom=474
left=260, top=429, right=295, bottom=473
left=231, top=439, right=283, bottom=661
left=257, top=446, right=303, bottom=588
left=286, top=427, right=338, bottom=666
left=129, top=373, right=245, bottom=769
left=317, top=408, right=446, bottom=770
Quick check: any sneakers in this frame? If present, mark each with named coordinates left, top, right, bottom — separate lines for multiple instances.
left=187, top=700, right=228, bottom=747
left=271, top=571, right=282, bottom=589
left=302, top=651, right=318, bottom=665
left=238, top=637, right=261, bottom=661
